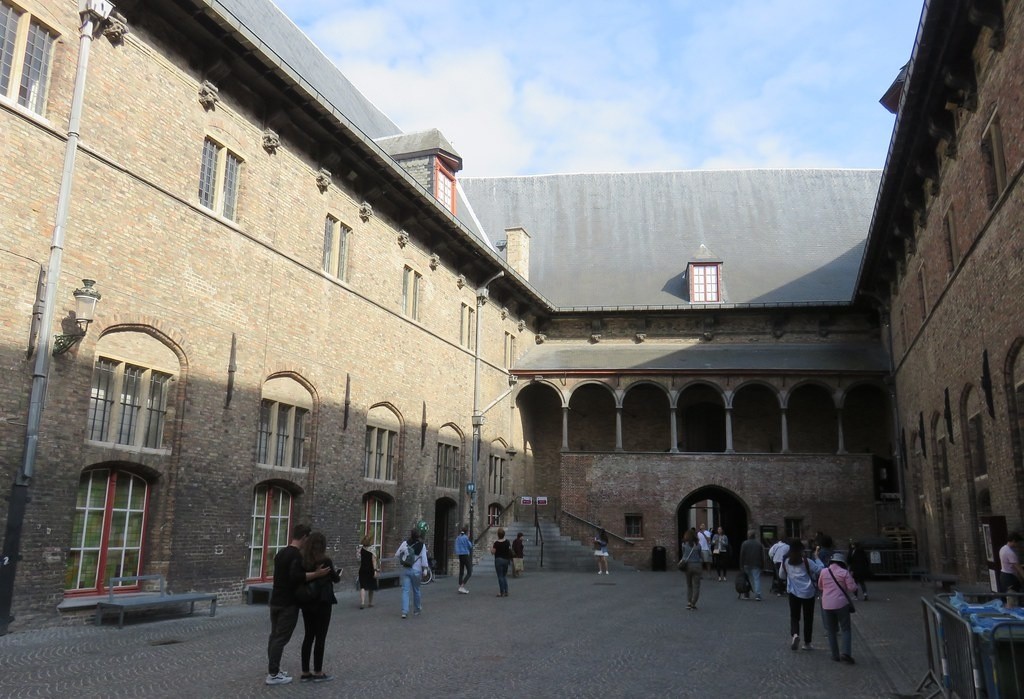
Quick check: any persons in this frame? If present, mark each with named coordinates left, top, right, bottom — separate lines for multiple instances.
left=266, top=524, right=331, bottom=685
left=847, top=540, right=869, bottom=601
left=681, top=528, right=702, bottom=610
left=813, top=531, right=833, bottom=568
left=768, top=536, right=791, bottom=597
left=358, top=534, right=380, bottom=609
left=817, top=553, right=860, bottom=663
left=711, top=526, right=729, bottom=582
left=511, top=532, right=524, bottom=578
left=297, top=532, right=342, bottom=683
left=999, top=533, right=1024, bottom=607
left=778, top=539, right=824, bottom=650
left=395, top=528, right=428, bottom=618
left=697, top=523, right=714, bottom=580
left=740, top=529, right=764, bottom=601
left=491, top=528, right=512, bottom=597
left=593, top=528, right=610, bottom=576
left=454, top=527, right=473, bottom=594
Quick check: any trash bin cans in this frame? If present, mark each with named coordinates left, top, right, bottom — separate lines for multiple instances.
left=652, top=546, right=667, bottom=571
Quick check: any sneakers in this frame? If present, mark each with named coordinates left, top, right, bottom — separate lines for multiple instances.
left=265, top=672, right=293, bottom=685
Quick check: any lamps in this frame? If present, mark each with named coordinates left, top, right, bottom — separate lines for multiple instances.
left=52, top=279, right=102, bottom=357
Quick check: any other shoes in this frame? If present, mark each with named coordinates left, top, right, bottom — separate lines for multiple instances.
left=802, top=643, right=814, bottom=650
left=458, top=587, right=469, bottom=594
left=830, top=654, right=856, bottom=664
left=414, top=608, right=422, bottom=615
left=313, top=673, right=334, bottom=682
left=401, top=614, right=408, bottom=617
left=755, top=596, right=762, bottom=601
left=851, top=596, right=858, bottom=601
left=368, top=603, right=374, bottom=607
left=863, top=594, right=868, bottom=601
left=685, top=604, right=698, bottom=611
left=300, top=672, right=314, bottom=682
left=742, top=596, right=749, bottom=600
left=497, top=591, right=509, bottom=597
left=598, top=570, right=602, bottom=575
left=606, top=570, right=609, bottom=575
left=791, top=636, right=800, bottom=651
left=359, top=604, right=365, bottom=609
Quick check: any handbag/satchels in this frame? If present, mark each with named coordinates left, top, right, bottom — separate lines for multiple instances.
left=513, top=557, right=525, bottom=572
left=676, top=559, right=687, bottom=572
left=713, top=548, right=720, bottom=554
left=510, top=549, right=516, bottom=558
left=847, top=602, right=856, bottom=613
left=593, top=541, right=602, bottom=550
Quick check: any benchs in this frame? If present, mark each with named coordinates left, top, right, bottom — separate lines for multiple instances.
left=374, top=571, right=401, bottom=589
left=247, top=583, right=274, bottom=606
left=95, top=575, right=218, bottom=630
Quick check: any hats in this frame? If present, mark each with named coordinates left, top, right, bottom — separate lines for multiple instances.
left=789, top=539, right=805, bottom=552
left=829, top=553, right=848, bottom=568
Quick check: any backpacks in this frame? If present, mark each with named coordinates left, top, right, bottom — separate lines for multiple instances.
left=400, top=541, right=422, bottom=568
left=735, top=570, right=751, bottom=593
